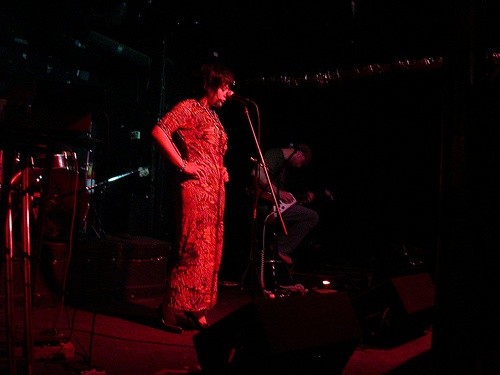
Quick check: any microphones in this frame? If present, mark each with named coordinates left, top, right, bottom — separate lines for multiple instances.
left=227, top=90, right=250, bottom=104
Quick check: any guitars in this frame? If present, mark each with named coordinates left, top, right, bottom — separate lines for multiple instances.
left=241, top=184, right=335, bottom=227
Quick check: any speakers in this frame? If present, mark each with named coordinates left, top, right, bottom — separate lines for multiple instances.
left=373, top=273, right=437, bottom=340
left=193, top=290, right=359, bottom=375
